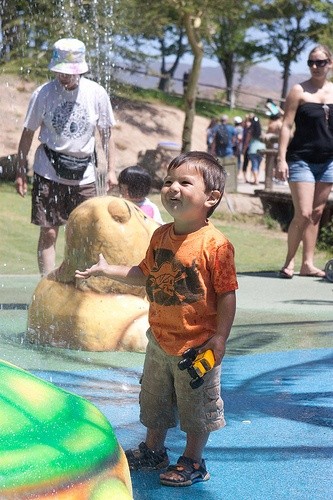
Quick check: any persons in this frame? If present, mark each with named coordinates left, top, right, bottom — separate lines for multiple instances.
left=74, top=151, right=239, bottom=487
left=14, top=39, right=119, bottom=278
left=277, top=46, right=333, bottom=278
left=207, top=98, right=289, bottom=185
left=117, top=166, right=164, bottom=227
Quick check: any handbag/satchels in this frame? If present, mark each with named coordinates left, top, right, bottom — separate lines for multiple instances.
left=207, top=134, right=215, bottom=148
left=43, top=144, right=91, bottom=180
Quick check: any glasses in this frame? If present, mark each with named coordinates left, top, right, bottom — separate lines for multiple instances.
left=308, top=59, right=328, bottom=67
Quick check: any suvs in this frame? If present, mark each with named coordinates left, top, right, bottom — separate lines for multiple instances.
left=178, top=346, right=216, bottom=390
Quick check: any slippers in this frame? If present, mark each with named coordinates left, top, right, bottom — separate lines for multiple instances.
left=280, top=267, right=293, bottom=278
left=301, top=270, right=326, bottom=277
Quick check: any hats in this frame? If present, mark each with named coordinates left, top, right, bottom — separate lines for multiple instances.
left=253, top=116, right=259, bottom=122
left=234, top=116, right=243, bottom=124
left=49, top=38, right=89, bottom=75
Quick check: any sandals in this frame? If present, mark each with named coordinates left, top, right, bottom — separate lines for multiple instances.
left=125, top=441, right=170, bottom=471
left=160, top=456, right=210, bottom=487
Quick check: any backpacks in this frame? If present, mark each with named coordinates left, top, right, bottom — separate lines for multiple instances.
left=216, top=124, right=229, bottom=147
